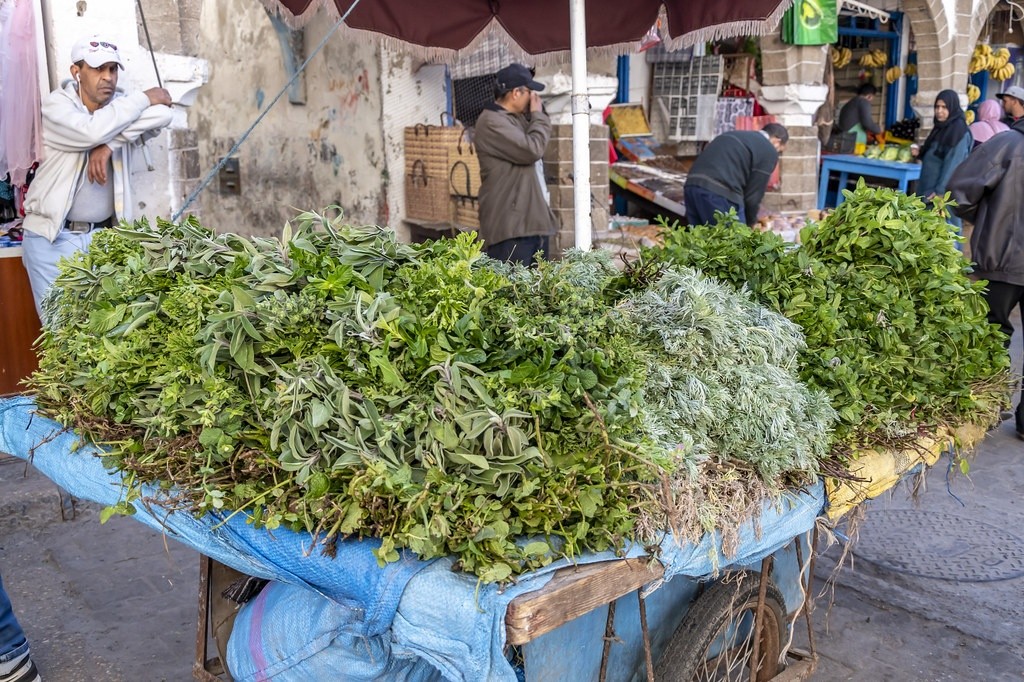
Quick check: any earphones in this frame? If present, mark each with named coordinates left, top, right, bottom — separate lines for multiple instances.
left=76, top=73, right=81, bottom=81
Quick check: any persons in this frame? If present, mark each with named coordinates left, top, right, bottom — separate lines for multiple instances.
left=970, top=101, right=1010, bottom=148
left=915, top=89, right=974, bottom=235
left=21, top=39, right=173, bottom=332
left=684, top=123, right=789, bottom=229
left=995, top=85, right=1024, bottom=127
left=945, top=118, right=1024, bottom=439
left=0, top=578, right=41, bottom=682
left=473, top=64, right=559, bottom=268
left=838, top=82, right=880, bottom=146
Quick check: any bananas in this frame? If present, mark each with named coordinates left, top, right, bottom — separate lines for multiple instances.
left=964, top=110, right=975, bottom=125
left=966, top=84, right=980, bottom=104
left=968, top=44, right=1015, bottom=80
left=859, top=49, right=887, bottom=67
left=885, top=64, right=917, bottom=83
left=830, top=47, right=852, bottom=68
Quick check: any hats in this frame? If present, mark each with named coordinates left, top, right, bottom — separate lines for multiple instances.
left=71, top=34, right=124, bottom=70
left=494, top=63, right=545, bottom=93
left=996, top=87, right=1024, bottom=101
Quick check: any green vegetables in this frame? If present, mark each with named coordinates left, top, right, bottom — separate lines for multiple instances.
left=17, top=175, right=1011, bottom=587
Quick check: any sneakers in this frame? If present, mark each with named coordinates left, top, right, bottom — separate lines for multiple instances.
left=0, top=654, right=42, bottom=682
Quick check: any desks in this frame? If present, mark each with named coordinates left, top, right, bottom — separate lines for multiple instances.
left=818, top=155, right=922, bottom=210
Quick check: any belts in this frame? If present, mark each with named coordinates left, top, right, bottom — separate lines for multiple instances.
left=63, top=218, right=110, bottom=231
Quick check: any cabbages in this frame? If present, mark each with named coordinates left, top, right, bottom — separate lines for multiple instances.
left=863, top=143, right=915, bottom=164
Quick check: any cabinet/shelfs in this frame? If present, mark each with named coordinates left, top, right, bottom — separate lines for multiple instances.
left=0, top=247, right=53, bottom=398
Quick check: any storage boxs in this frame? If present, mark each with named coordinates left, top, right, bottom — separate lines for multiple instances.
left=607, top=103, right=655, bottom=140
left=615, top=136, right=658, bottom=161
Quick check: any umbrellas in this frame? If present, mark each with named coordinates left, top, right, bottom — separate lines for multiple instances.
left=258, top=0, right=791, bottom=248
